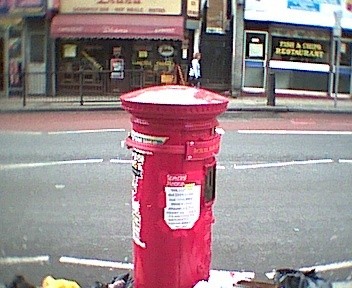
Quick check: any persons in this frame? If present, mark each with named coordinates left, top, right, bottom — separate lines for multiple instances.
left=187, top=51, right=201, bottom=86
